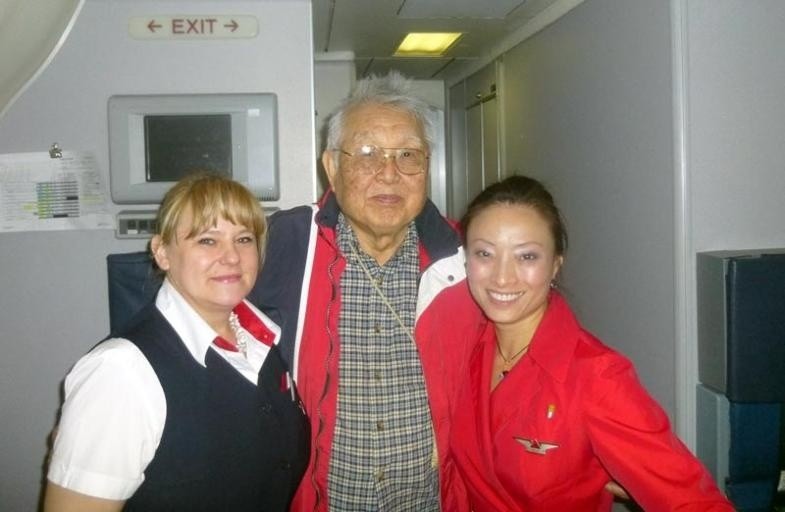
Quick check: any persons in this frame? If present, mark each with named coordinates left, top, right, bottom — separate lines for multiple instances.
left=248, top=74, right=488, bottom=510
left=446, top=176, right=731, bottom=511
left=42, top=176, right=310, bottom=512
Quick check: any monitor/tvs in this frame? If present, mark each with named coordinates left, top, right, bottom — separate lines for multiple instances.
left=110, top=94, right=280, bottom=205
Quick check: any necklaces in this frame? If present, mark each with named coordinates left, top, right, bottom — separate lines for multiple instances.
left=495, top=336, right=528, bottom=378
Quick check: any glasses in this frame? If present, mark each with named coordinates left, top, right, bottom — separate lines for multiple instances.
left=336, top=145, right=430, bottom=176
